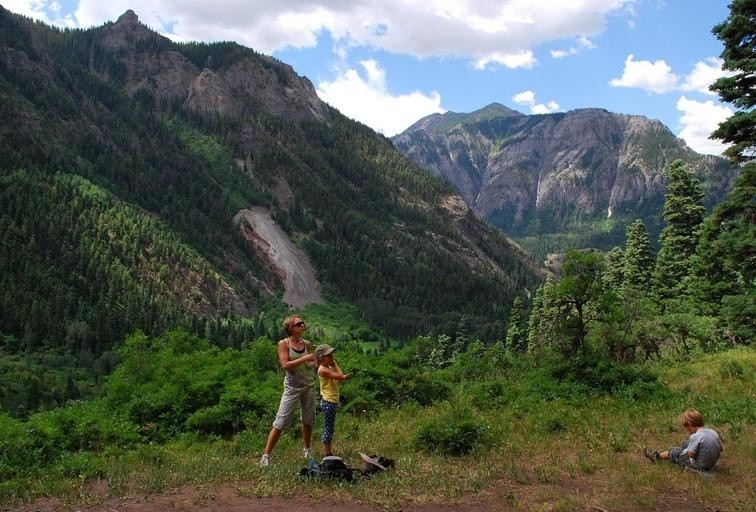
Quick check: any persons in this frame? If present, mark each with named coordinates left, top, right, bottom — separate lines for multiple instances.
left=641, top=407, right=725, bottom=469
left=258, top=314, right=320, bottom=470
left=313, top=343, right=353, bottom=461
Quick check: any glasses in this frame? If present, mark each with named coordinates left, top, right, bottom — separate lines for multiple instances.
left=292, top=322, right=304, bottom=327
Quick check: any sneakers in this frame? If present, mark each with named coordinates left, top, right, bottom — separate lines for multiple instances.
left=644, top=447, right=658, bottom=464
left=304, top=448, right=313, bottom=461
left=260, top=454, right=270, bottom=468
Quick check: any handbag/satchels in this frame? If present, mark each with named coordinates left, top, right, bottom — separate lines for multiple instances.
left=306, top=456, right=353, bottom=483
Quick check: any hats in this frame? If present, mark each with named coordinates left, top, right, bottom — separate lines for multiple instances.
left=358, top=452, right=387, bottom=474
left=314, top=344, right=336, bottom=356
